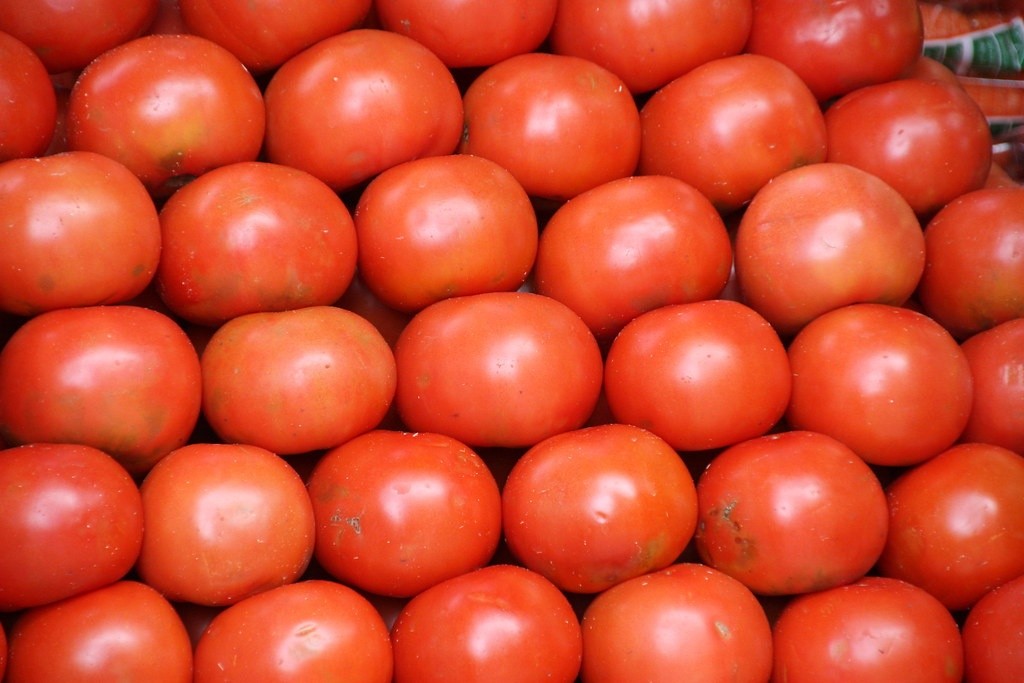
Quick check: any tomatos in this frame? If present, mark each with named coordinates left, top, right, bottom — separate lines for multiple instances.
left=0, top=0, right=1024, bottom=682
